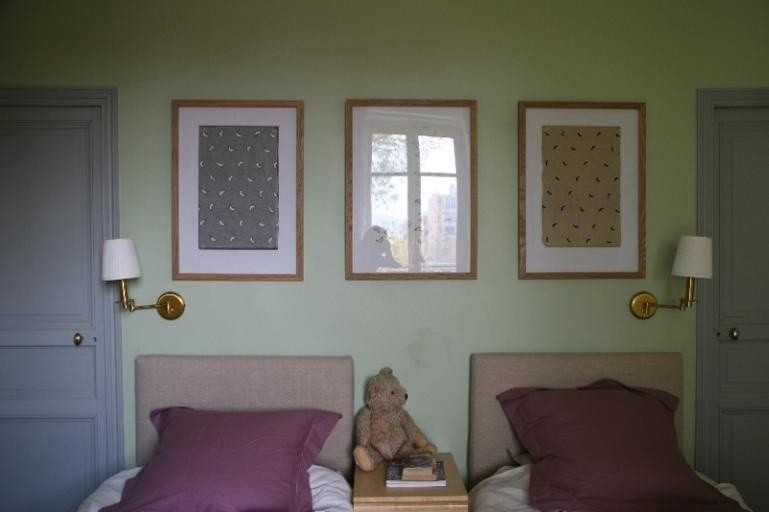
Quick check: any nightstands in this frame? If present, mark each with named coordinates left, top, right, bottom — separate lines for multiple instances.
left=353, top=454, right=470, bottom=512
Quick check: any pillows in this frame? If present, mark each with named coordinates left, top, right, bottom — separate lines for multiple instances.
left=96, top=405, right=342, bottom=512
left=495, top=378, right=747, bottom=512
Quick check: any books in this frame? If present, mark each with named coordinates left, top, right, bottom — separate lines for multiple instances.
left=386, top=458, right=446, bottom=488
left=401, top=453, right=436, bottom=480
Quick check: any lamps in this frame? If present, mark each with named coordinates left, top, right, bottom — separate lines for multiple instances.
left=629, top=236, right=714, bottom=320
left=101, top=238, right=185, bottom=320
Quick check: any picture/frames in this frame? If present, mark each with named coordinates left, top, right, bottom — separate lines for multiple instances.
left=171, top=100, right=303, bottom=281
left=518, top=101, right=646, bottom=280
left=345, top=99, right=478, bottom=280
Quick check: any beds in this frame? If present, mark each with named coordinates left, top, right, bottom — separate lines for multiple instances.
left=467, top=353, right=752, bottom=512
left=78, top=356, right=353, bottom=512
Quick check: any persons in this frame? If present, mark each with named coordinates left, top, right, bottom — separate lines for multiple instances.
left=357, top=226, right=401, bottom=272
